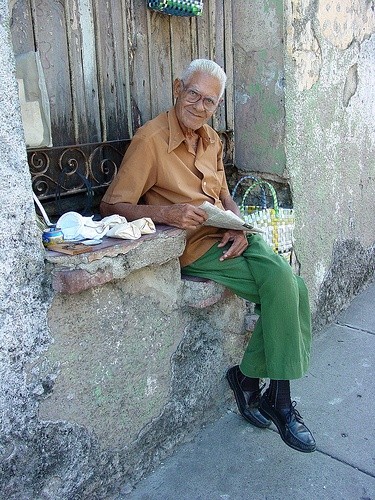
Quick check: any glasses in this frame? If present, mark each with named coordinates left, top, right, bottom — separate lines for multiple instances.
left=181, top=79, right=220, bottom=113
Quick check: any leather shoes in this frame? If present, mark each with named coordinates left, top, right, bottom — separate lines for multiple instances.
left=257, top=392, right=317, bottom=453
left=225, top=365, right=272, bottom=427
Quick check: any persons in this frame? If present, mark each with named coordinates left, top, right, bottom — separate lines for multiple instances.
left=100, top=59, right=316, bottom=453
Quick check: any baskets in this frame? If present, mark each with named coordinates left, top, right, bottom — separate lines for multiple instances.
left=231, top=175, right=294, bottom=268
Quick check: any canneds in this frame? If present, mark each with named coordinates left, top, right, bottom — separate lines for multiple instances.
left=42, top=225, right=64, bottom=251
